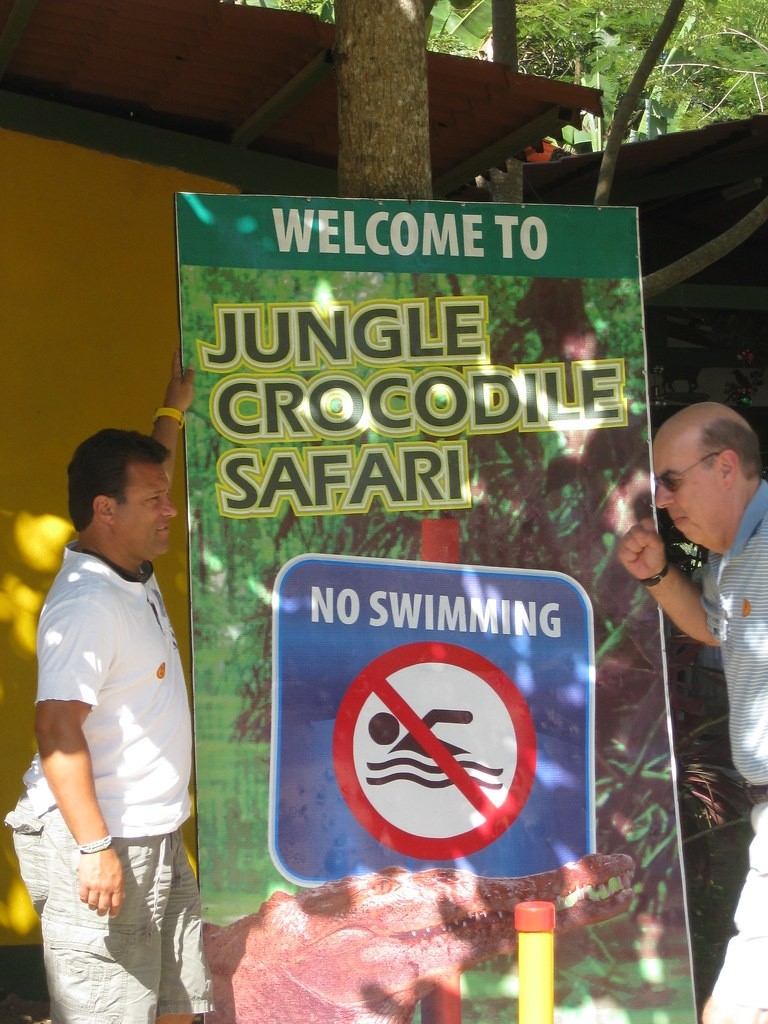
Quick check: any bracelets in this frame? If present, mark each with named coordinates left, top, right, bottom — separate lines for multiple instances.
left=639, top=559, right=668, bottom=586
left=77, top=835, right=113, bottom=854
left=153, top=407, right=186, bottom=430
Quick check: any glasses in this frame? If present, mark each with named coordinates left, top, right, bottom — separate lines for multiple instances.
left=653, top=452, right=719, bottom=493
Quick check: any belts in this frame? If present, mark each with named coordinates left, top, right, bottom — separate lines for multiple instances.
left=746, top=785, right=768, bottom=804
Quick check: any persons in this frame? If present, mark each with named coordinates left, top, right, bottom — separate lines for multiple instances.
left=617, top=401, right=767, bottom=1024
left=4, top=348, right=215, bottom=1024
